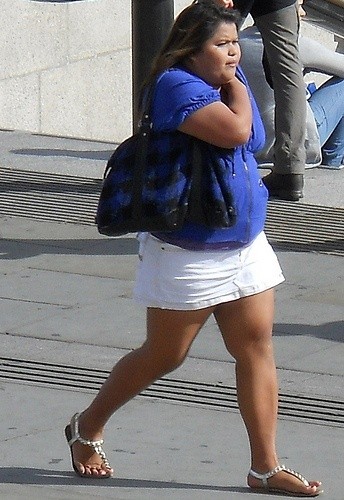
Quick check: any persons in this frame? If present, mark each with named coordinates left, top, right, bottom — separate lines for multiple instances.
left=65, top=0, right=325, bottom=498
left=191, top=0, right=307, bottom=201
left=237, top=0, right=344, bottom=171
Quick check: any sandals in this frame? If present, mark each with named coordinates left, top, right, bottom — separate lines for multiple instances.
left=65, top=412, right=114, bottom=478
left=247, top=464, right=324, bottom=496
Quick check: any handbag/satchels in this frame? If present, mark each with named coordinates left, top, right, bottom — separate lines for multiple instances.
left=95, top=130, right=237, bottom=236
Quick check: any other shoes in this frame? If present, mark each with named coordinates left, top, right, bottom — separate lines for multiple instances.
left=318, top=164, right=344, bottom=169
left=261, top=171, right=304, bottom=201
